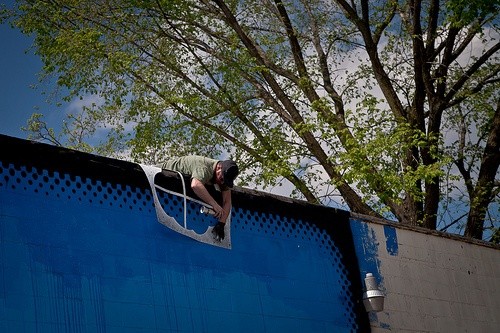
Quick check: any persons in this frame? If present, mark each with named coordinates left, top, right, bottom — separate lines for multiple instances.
left=152, top=155, right=239, bottom=243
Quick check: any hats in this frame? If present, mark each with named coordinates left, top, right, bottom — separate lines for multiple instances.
left=222, top=160, right=239, bottom=189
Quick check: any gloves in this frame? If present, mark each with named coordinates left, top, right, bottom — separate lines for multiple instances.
left=212, top=221, right=226, bottom=242
